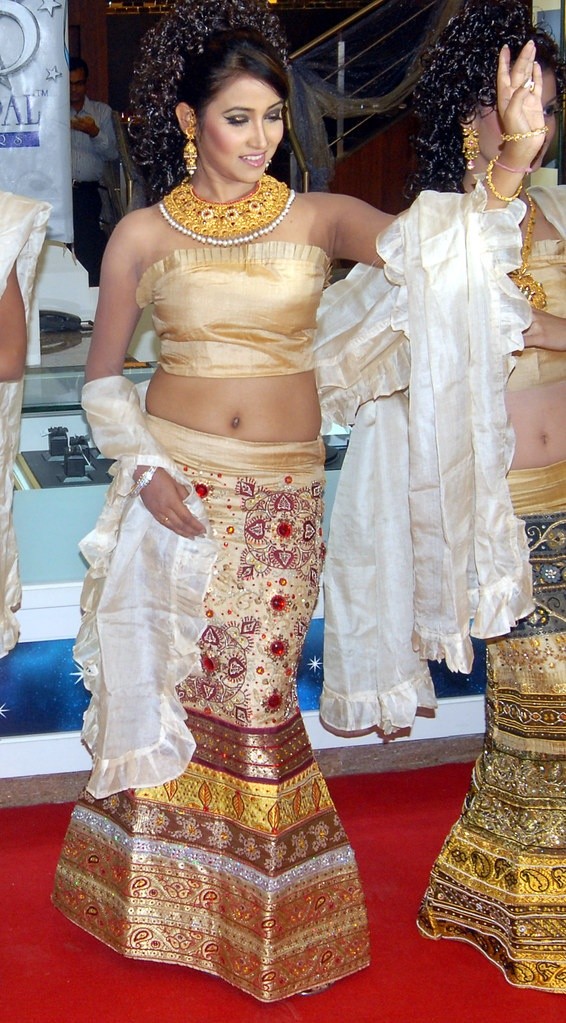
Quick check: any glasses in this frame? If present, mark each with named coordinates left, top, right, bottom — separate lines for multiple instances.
left=70, top=81, right=87, bottom=88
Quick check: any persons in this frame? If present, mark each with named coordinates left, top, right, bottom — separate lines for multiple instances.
left=70, top=58, right=120, bottom=253
left=1, top=188, right=54, bottom=657
left=402, top=0, right=566, bottom=992
left=50, top=0, right=546, bottom=999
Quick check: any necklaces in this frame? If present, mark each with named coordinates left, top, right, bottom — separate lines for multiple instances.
left=160, top=174, right=296, bottom=247
left=507, top=188, right=547, bottom=309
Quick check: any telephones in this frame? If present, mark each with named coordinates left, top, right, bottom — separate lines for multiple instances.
left=39, top=310, right=82, bottom=332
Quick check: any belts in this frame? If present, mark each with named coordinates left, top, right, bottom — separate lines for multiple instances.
left=72, top=178, right=100, bottom=189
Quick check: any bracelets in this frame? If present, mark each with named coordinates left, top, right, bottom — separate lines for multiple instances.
left=486, top=155, right=522, bottom=202
left=129, top=466, right=159, bottom=498
left=495, top=161, right=532, bottom=173
left=501, top=125, right=549, bottom=142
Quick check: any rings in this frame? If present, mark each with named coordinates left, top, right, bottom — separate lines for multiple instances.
left=164, top=518, right=169, bottom=522
left=528, top=83, right=535, bottom=92
